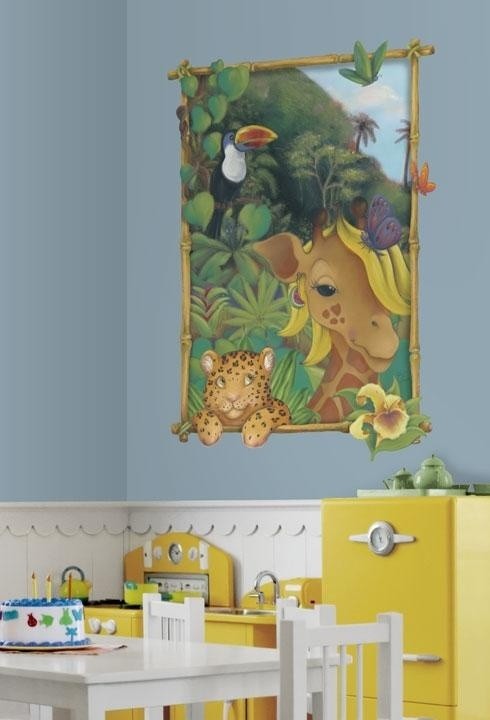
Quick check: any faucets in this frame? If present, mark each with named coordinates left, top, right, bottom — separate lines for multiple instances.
left=253, top=570, right=281, bottom=606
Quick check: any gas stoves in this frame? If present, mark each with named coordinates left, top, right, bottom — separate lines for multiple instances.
left=82, top=598, right=145, bottom=638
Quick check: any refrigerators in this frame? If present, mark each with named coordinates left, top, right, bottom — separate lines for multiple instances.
left=317, top=498, right=489, bottom=720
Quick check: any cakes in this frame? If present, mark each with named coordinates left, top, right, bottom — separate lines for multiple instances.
left=0, top=597, right=91, bottom=646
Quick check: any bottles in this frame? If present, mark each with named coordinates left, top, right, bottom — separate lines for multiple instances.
left=393, top=467, right=414, bottom=492
left=414, top=455, right=452, bottom=488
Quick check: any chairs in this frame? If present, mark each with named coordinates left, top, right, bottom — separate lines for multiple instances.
left=272, top=595, right=345, bottom=712
left=136, top=591, right=234, bottom=719
left=275, top=611, right=413, bottom=718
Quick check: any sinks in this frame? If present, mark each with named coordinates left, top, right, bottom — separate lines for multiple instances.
left=204, top=606, right=277, bottom=616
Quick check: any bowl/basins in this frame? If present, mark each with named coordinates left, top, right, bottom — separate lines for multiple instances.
left=170, top=591, right=204, bottom=602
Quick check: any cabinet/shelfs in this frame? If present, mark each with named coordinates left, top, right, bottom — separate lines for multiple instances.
left=70, top=603, right=283, bottom=719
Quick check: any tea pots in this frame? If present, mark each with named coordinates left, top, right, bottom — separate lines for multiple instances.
left=59, top=566, right=92, bottom=602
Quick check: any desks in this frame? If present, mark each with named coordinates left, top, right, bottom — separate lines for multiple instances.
left=1, top=633, right=354, bottom=719
